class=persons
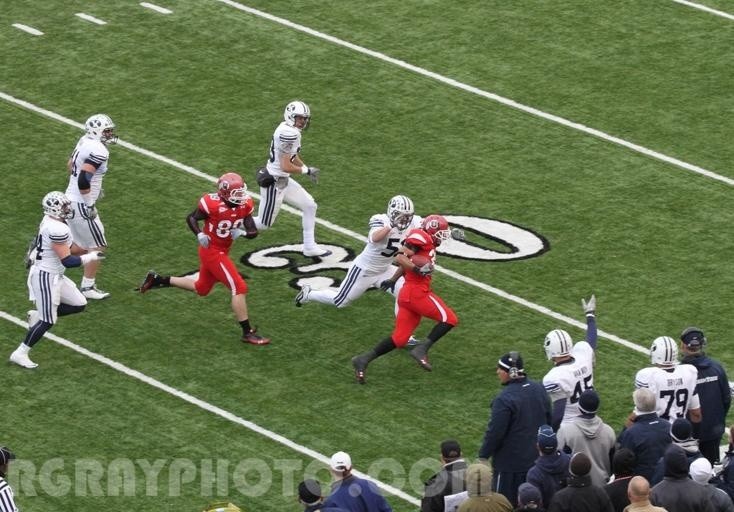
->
[350,214,459,384]
[24,113,118,300]
[237,101,326,257]
[138,173,271,345]
[9,191,106,369]
[294,195,464,346]
[2,291,732,511]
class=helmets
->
[386,195,415,230]
[650,336,679,366]
[544,329,575,361]
[284,100,312,131]
[218,172,248,206]
[85,113,119,146]
[421,214,451,247]
[41,191,75,219]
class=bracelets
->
[389,223,393,229]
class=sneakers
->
[294,283,312,307]
[240,325,271,344]
[10,351,39,369]
[80,282,111,300]
[139,270,158,293]
[351,332,432,385]
[302,246,327,256]
[27,310,39,330]
[23,236,38,269]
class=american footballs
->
[409,256,434,274]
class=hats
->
[330,450,351,472]
[669,418,693,442]
[689,457,712,486]
[680,326,704,348]
[497,354,525,373]
[440,390,599,510]
[298,479,321,503]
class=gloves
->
[84,205,99,220]
[90,250,106,260]
[451,228,466,241]
[197,232,211,248]
[380,279,396,294]
[417,261,434,277]
[388,212,404,228]
[98,188,106,201]
[311,175,319,185]
[229,228,247,240]
[581,294,597,317]
[307,167,320,176]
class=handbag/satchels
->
[255,167,275,187]
